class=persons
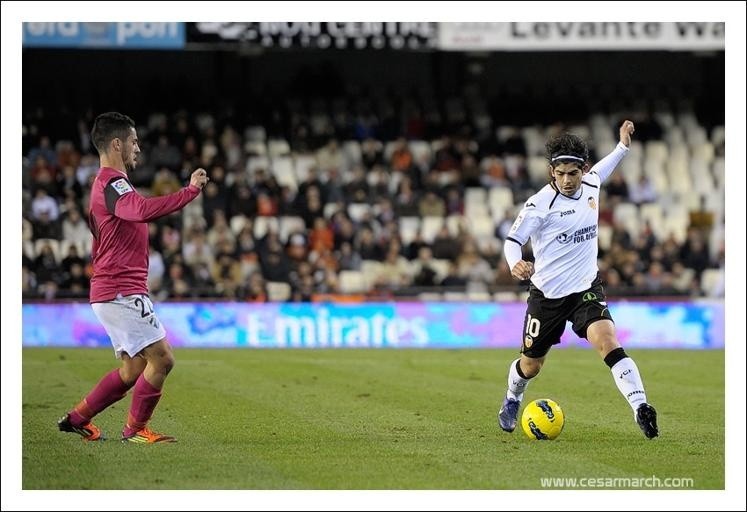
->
[56,112,211,446]
[496,113,661,441]
[22,92,724,304]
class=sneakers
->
[57,413,108,441]
[498,388,520,432]
[636,403,658,439]
[121,427,177,445]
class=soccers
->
[522,398,564,441]
